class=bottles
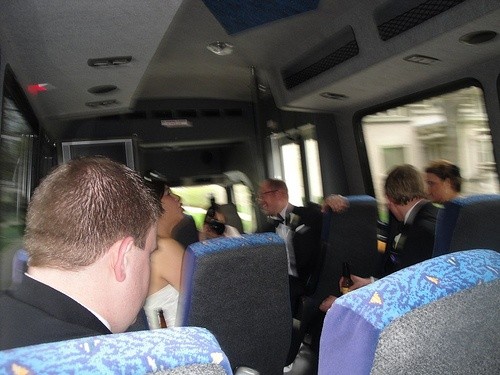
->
[152,307,167,329]
[341,261,353,294]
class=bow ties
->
[272,213,284,228]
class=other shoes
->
[283,343,317,375]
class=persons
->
[1,151,167,351]
[142,161,465,375]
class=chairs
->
[0,326,233,375]
[431,194,500,256]
[174,232,315,375]
[318,247,500,375]
[311,194,377,300]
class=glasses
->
[255,190,278,201]
